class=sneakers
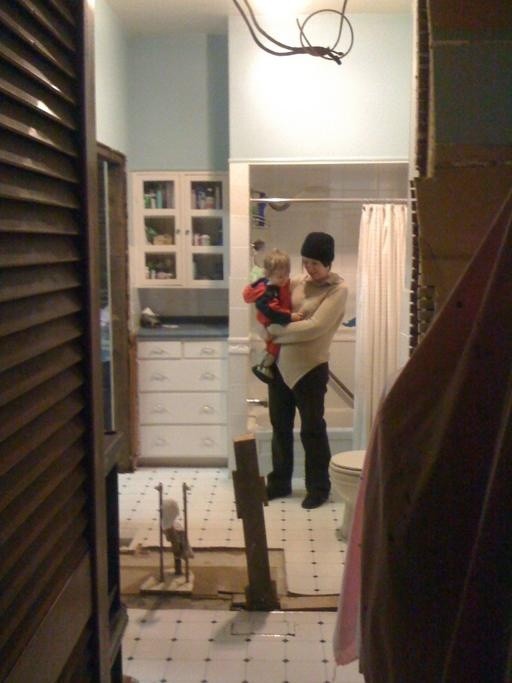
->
[252,365,274,384]
[266,485,328,508]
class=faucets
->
[247,398,269,407]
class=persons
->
[238,247,307,385]
[264,231,352,510]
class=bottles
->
[144,193,156,208]
[206,187,214,209]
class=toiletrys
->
[143,186,215,281]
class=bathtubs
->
[250,406,356,476]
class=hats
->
[301,232,334,268]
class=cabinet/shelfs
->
[134,338,228,468]
[128,169,229,288]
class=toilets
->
[328,450,369,533]
